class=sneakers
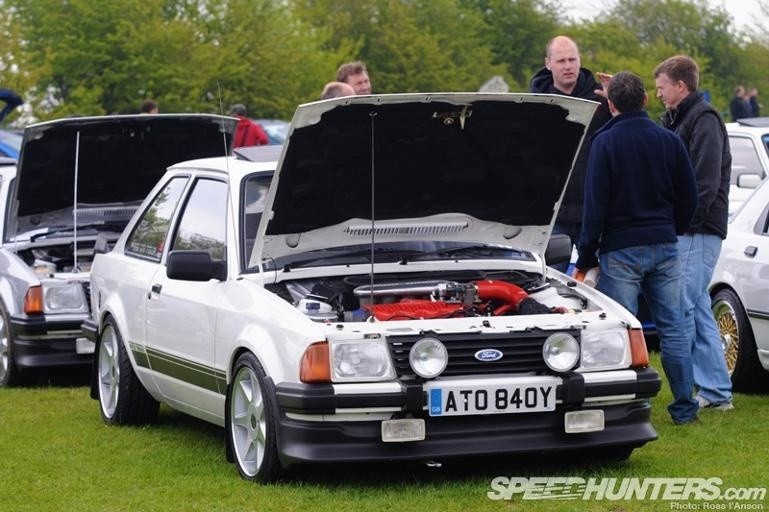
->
[694,393,735,413]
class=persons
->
[140,100,158,114]
[572,71,699,423]
[226,103,269,149]
[321,81,356,100]
[729,85,751,122]
[336,60,371,96]
[529,35,614,278]
[749,88,763,117]
[653,56,735,410]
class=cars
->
[0,87,26,162]
[715,115,769,232]
[80,84,670,471]
[251,118,296,148]
[704,172,768,402]
[0,108,246,392]
